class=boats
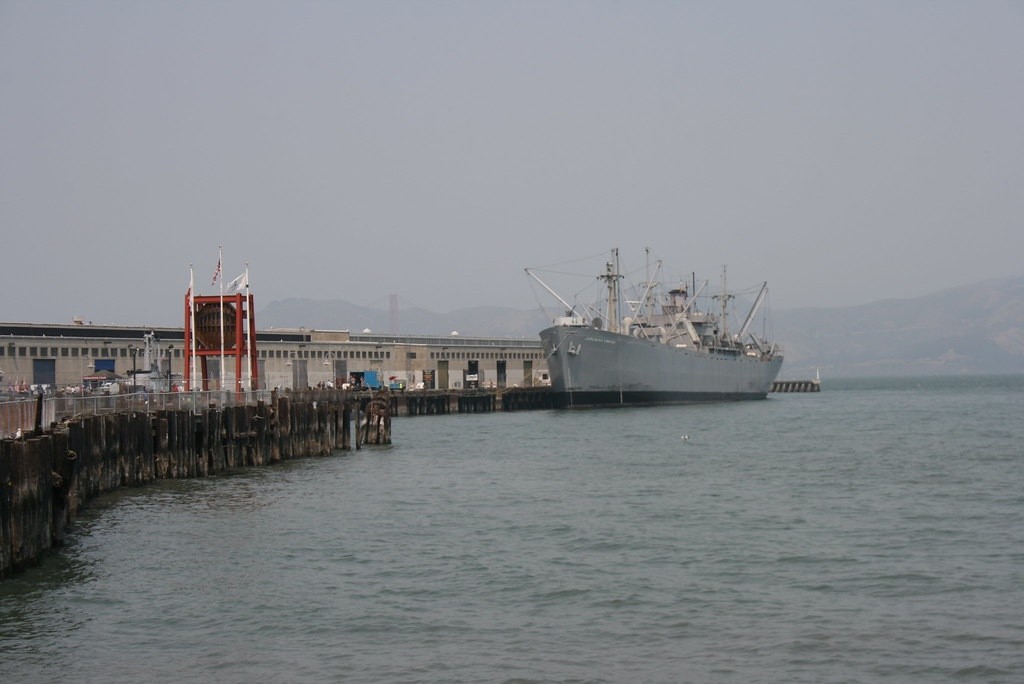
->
[522,248,789,408]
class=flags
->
[185,280,192,312]
[212,258,220,285]
[227,273,247,291]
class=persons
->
[61,385,80,394]
[316,379,334,390]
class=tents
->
[84,369,124,386]
